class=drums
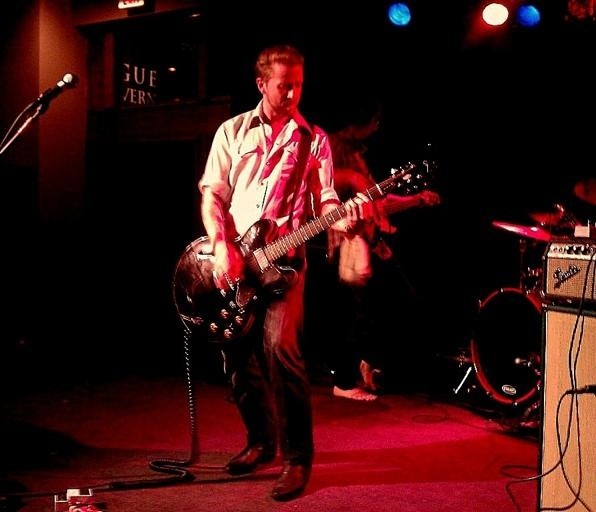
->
[471,287,543,405]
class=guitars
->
[171,160,437,345]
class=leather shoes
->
[271,461,312,500]
[224,436,277,473]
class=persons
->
[321,113,442,406]
[195,42,372,502]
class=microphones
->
[24,73,79,112]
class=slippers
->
[360,362,377,389]
[333,388,378,402]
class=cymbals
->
[489,220,569,245]
[531,212,584,231]
